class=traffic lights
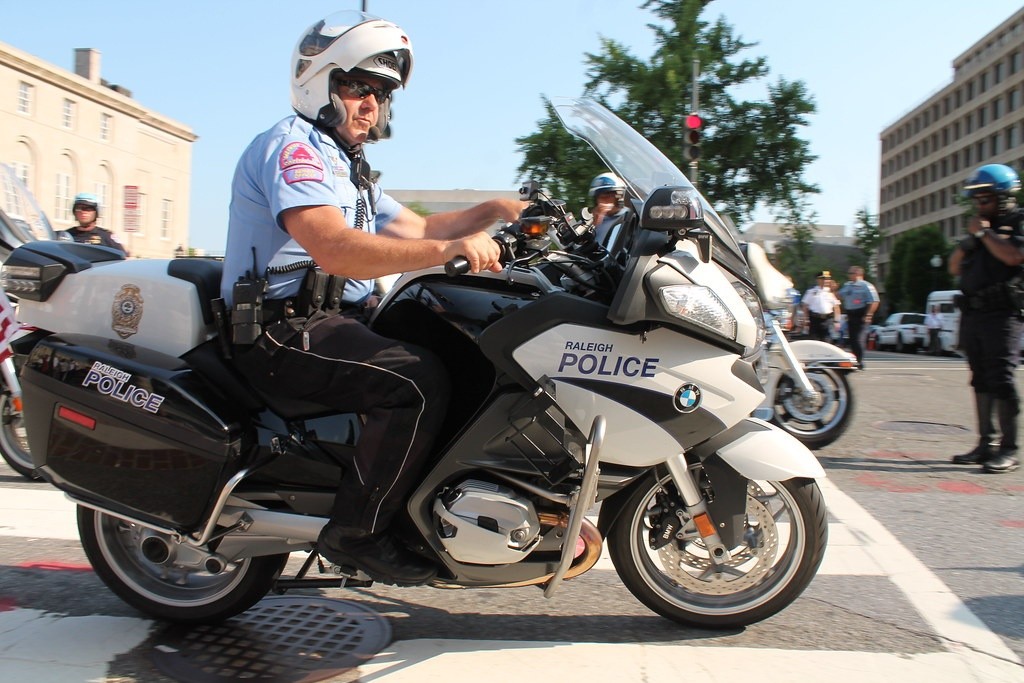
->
[684,111,703,160]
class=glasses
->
[971,196,992,205]
[337,79,387,104]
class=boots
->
[953,393,1002,464]
[984,394,1024,474]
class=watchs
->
[974,227,993,238]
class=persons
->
[782,274,800,341]
[801,270,841,344]
[836,266,881,369]
[586,173,627,230]
[947,163,1024,473]
[221,10,535,588]
[53,193,129,257]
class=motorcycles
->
[1,93,831,638]
[1,163,74,483]
[717,211,860,452]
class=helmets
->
[73,195,102,219]
[589,173,625,208]
[964,164,1022,196]
[291,11,412,140]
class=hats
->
[815,271,832,279]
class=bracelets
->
[832,320,841,323]
[865,313,873,316]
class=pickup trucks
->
[869,311,933,353]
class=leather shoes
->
[319,520,438,588]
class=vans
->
[925,290,964,354]
